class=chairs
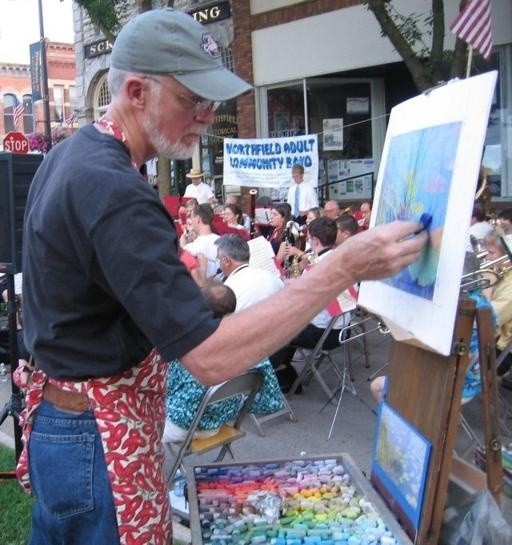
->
[163,370,266,490]
[249,306,512,470]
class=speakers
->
[0,152,43,273]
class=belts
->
[41,384,90,413]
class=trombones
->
[339,250,512,345]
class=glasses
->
[141,75,222,112]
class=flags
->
[449,0,493,63]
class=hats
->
[112,8,253,101]
[186,168,203,178]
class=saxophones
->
[293,248,312,279]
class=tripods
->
[0,273,26,467]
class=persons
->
[11,5,428,545]
